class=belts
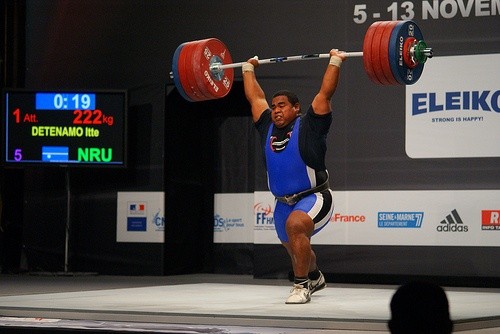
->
[276,179,330,206]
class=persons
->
[242,49,348,305]
[387,279,455,334]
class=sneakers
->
[285,283,311,304]
[309,270,327,295]
